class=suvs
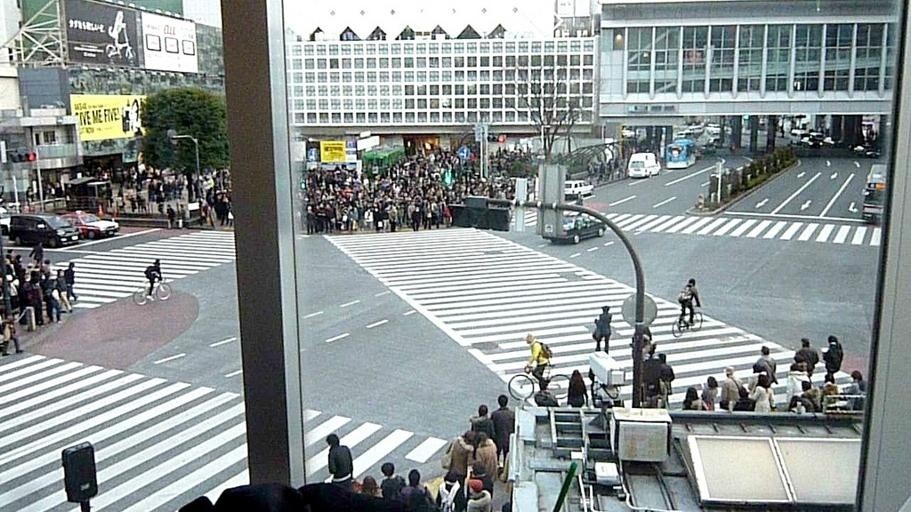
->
[564,180,593,199]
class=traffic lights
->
[10,150,36,162]
[487,134,506,143]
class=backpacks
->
[678,285,694,303]
[535,340,553,359]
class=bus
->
[665,139,695,170]
[863,164,887,225]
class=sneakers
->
[145,293,155,303]
[19,317,62,327]
[3,349,25,357]
[59,295,78,314]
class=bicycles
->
[671,303,703,338]
[132,275,171,306]
[507,364,571,401]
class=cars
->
[0,207,11,236]
[61,210,119,240]
[542,215,606,245]
[791,129,880,159]
[674,123,721,153]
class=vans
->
[627,152,661,179]
[8,214,80,249]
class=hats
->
[723,365,736,376]
[467,477,484,492]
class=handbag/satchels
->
[377,220,383,228]
[440,451,452,471]
[733,399,756,411]
[592,330,603,343]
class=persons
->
[0,241,78,356]
[303,145,531,235]
[682,336,868,415]
[678,278,701,324]
[125,100,142,136]
[638,336,675,409]
[326,433,493,511]
[446,395,515,483]
[144,259,162,301]
[525,334,588,407]
[28,165,234,229]
[595,306,612,353]
